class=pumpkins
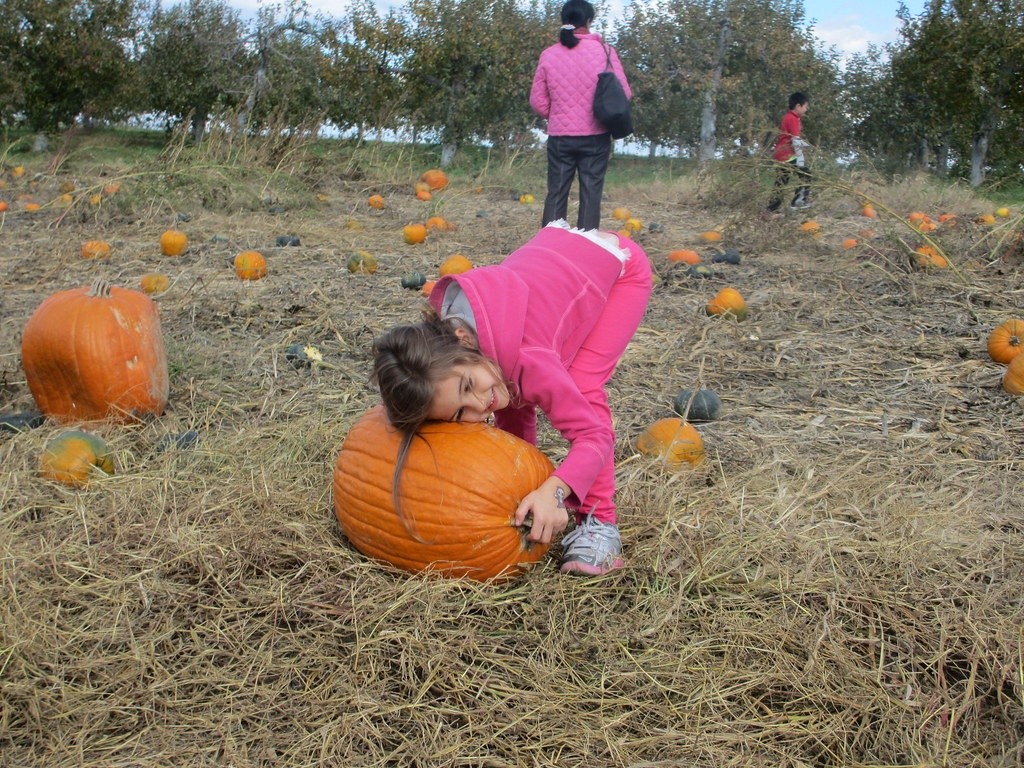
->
[0,168,1024,587]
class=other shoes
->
[769,210,787,220]
[791,201,811,211]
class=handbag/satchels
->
[592,41,635,141]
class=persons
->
[371,219,653,576]
[762,92,810,222]
[530,0,633,232]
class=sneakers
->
[559,500,625,577]
